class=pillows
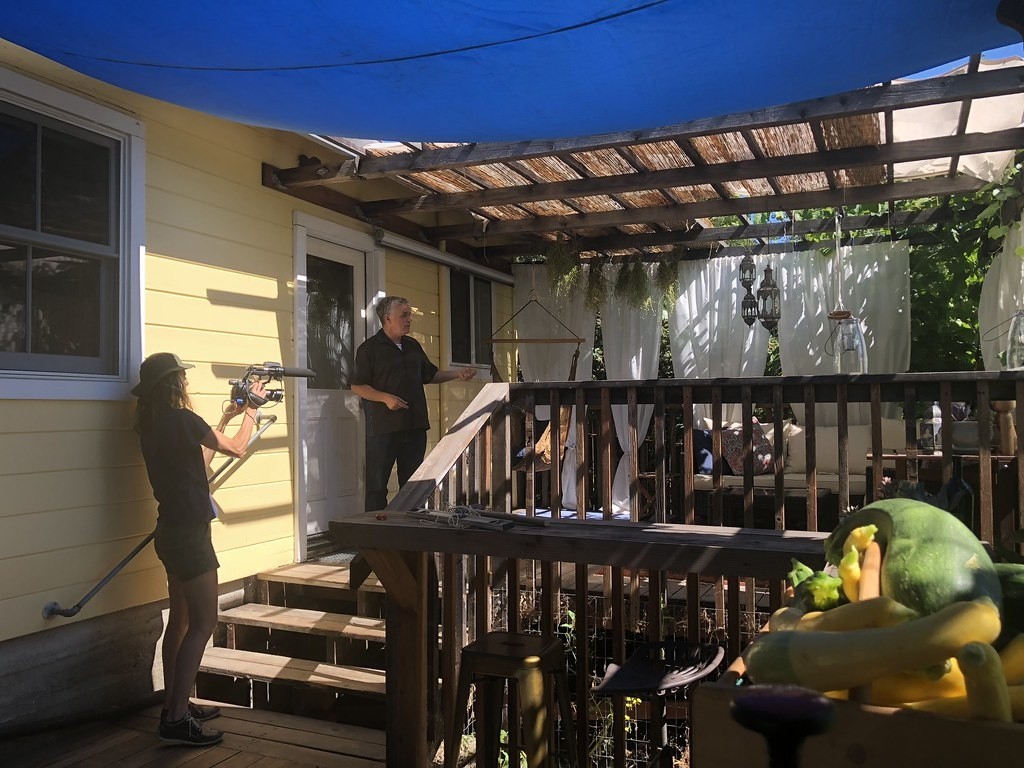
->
[785,424,872,475]
[880,417,920,468]
[691,425,731,475]
[709,415,778,475]
[760,419,793,462]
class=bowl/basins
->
[950,421,992,452]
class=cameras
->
[229,380,284,406]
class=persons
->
[346,296,477,512]
[131,353,269,746]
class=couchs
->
[695,417,932,531]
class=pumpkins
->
[738,498,1024,726]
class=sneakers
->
[160,700,220,720]
[157,711,224,745]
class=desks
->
[637,470,679,522]
[865,451,1019,559]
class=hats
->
[131,353,196,399]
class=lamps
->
[741,287,757,328]
[738,248,760,289]
[758,263,780,332]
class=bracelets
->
[245,411,255,422]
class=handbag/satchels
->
[982,310,1024,369]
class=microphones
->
[281,367,317,377]
